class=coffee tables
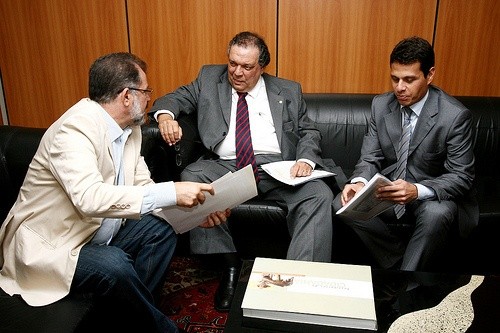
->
[223,259,500,333]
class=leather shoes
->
[214,262,241,312]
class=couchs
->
[0,122,139,333]
[141,91,500,271]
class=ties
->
[235,91,260,186]
[392,105,413,219]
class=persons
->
[332,37,479,272]
[0,52,231,333]
[146,32,347,313]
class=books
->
[335,173,398,226]
[241,258,377,330]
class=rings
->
[304,171,308,172]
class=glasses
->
[117,87,153,97]
